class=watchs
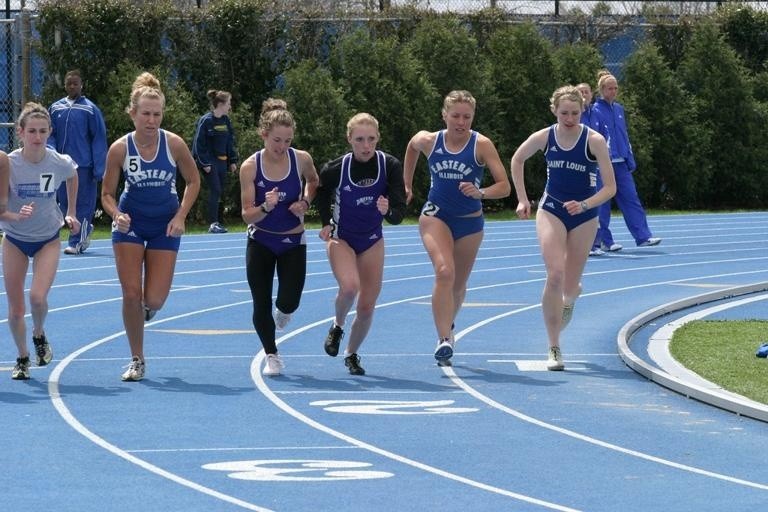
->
[581,200,589,212]
[479,189,486,199]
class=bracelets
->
[259,202,273,215]
[302,199,310,209]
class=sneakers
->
[64,223,94,253]
[557,283,582,332]
[121,360,145,381]
[208,223,227,234]
[263,352,280,376]
[325,322,343,357]
[344,354,365,375]
[32,331,52,366]
[275,309,292,328]
[12,357,30,379]
[548,345,564,371]
[590,237,661,256]
[142,303,157,321]
[435,322,454,366]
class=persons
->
[191,89,238,234]
[0,150,9,215]
[239,97,320,376]
[576,84,622,255]
[589,70,661,251]
[101,71,201,382]
[404,90,511,367]
[510,85,617,371]
[0,101,81,380]
[44,70,107,254]
[315,113,407,375]
